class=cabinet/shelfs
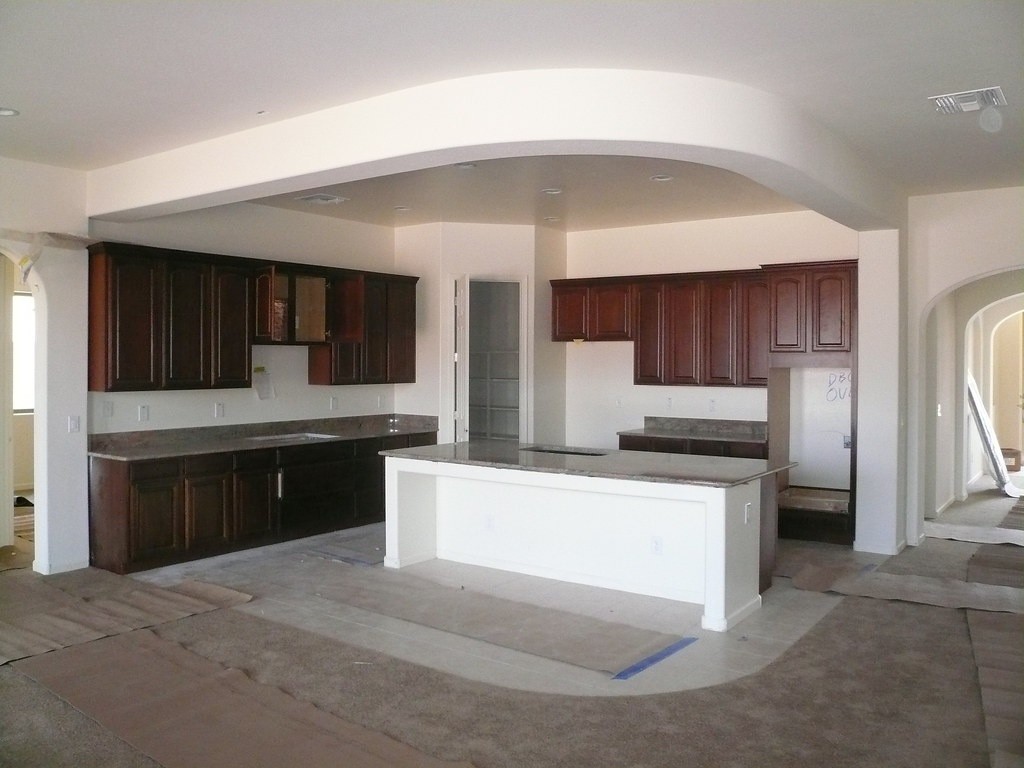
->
[79,239,417,392]
[86,432,437,575]
[618,434,769,461]
[547,257,860,390]
